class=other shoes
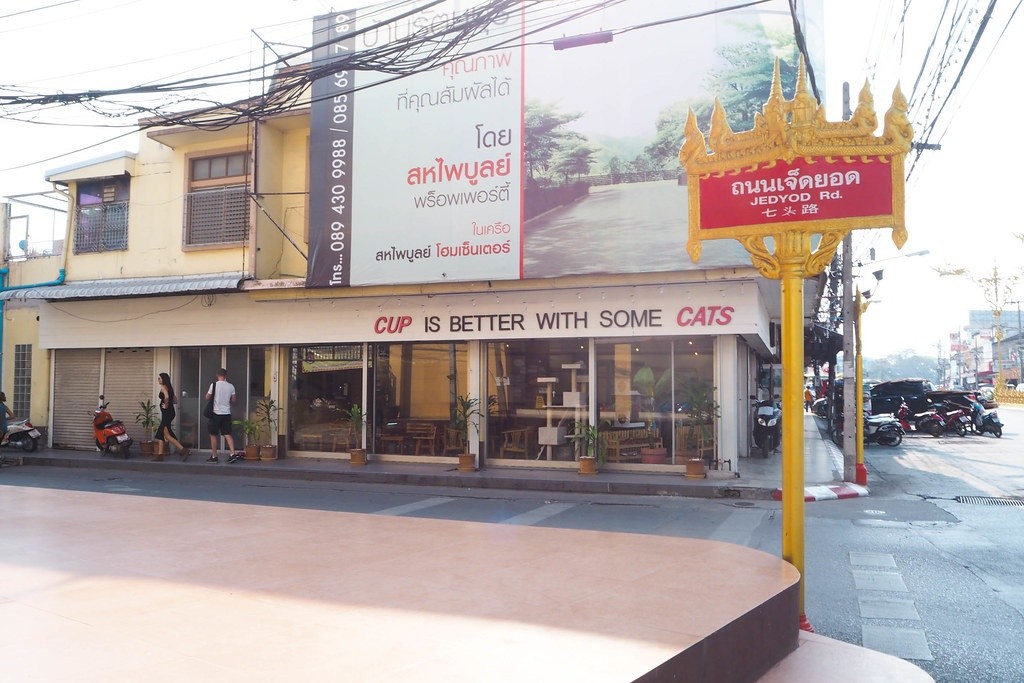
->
[151,453,164,461]
[180,448,191,461]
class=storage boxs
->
[562,391,586,406]
[538,427,567,445]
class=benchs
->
[598,427,663,463]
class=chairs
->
[411,425,438,455]
[301,434,323,451]
[498,425,543,459]
[379,418,405,456]
[329,424,352,453]
[443,425,466,457]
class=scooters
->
[0,417,42,453]
[86,395,133,458]
[750,393,782,458]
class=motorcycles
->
[805,377,1005,447]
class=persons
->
[205,368,239,464]
[0,392,15,443]
[150,373,190,463]
[805,386,814,413]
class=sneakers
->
[225,454,238,463]
[206,456,218,464]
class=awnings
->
[1,272,251,302]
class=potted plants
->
[675,385,723,481]
[568,415,613,475]
[131,398,161,456]
[229,414,265,462]
[253,393,284,462]
[448,391,485,472]
[151,415,184,456]
[626,363,672,464]
[347,403,369,465]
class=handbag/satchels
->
[204,382,216,418]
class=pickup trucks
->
[870,378,975,422]
